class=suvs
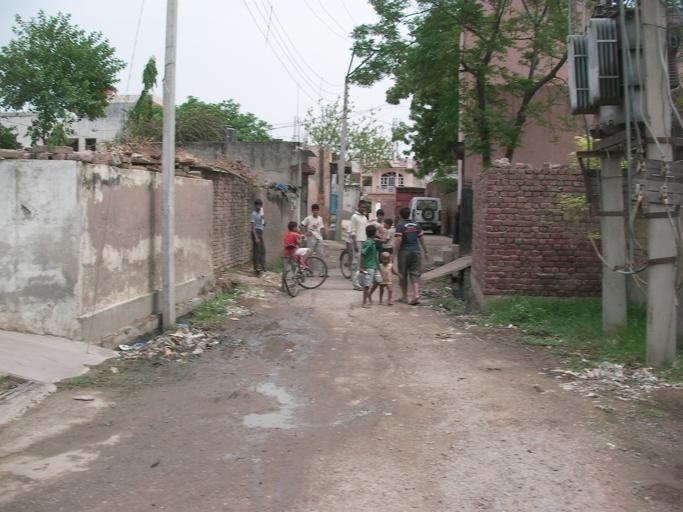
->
[405,197,443,236]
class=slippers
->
[394,298,420,305]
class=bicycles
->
[278,237,394,299]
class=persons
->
[248,198,269,276]
[299,203,330,276]
[277,221,311,293]
[345,199,430,308]
